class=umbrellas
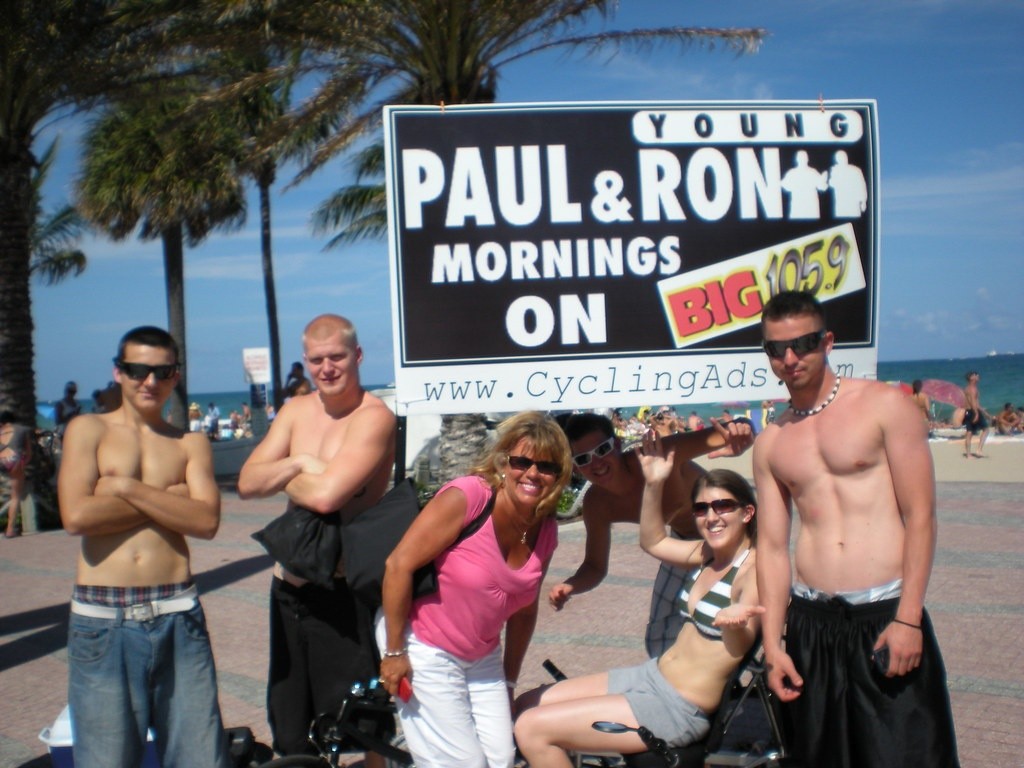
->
[919,379,970,422]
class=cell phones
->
[397,677,413,703]
[874,647,890,673]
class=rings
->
[379,678,385,683]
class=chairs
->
[571,598,789,768]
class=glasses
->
[114,358,179,380]
[505,454,561,474]
[571,437,615,468]
[692,498,747,517]
[761,328,829,359]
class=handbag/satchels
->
[341,476,499,598]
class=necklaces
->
[787,375,842,416]
[500,495,541,544]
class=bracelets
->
[384,647,408,658]
[506,680,517,689]
[893,617,921,630]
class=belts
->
[70,583,197,620]
[271,559,310,586]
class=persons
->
[378,412,573,768]
[752,292,959,768]
[598,377,1024,455]
[0,412,33,539]
[962,370,989,458]
[514,430,767,768]
[58,326,228,768]
[237,315,397,768]
[55,362,316,442]
[548,413,759,666]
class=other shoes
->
[5,530,19,537]
